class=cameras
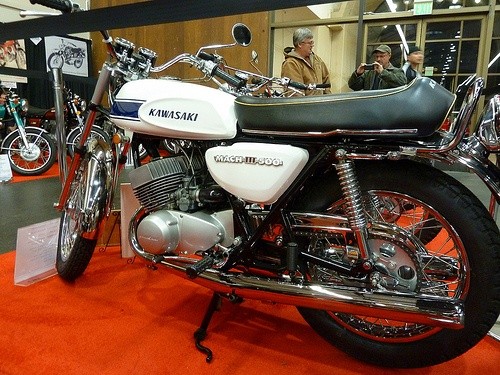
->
[364,64,374,70]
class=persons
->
[402,46,424,85]
[348,45,407,91]
[281,28,331,98]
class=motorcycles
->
[0,83,57,176]
[19,0,500,370]
[18,84,113,164]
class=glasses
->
[301,41,315,45]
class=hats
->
[406,46,421,56]
[372,45,391,53]
